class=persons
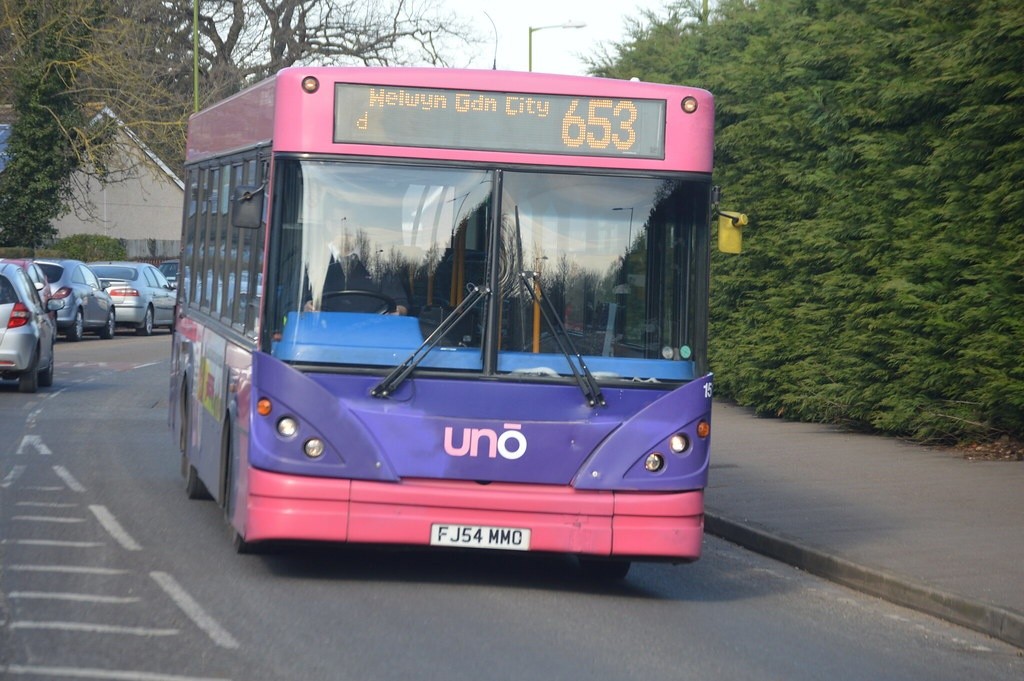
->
[302,205,409,316]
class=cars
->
[162,258,264,326]
[35,258,116,343]
[1,262,66,394]
[85,260,177,337]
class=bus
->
[169,64,750,586]
